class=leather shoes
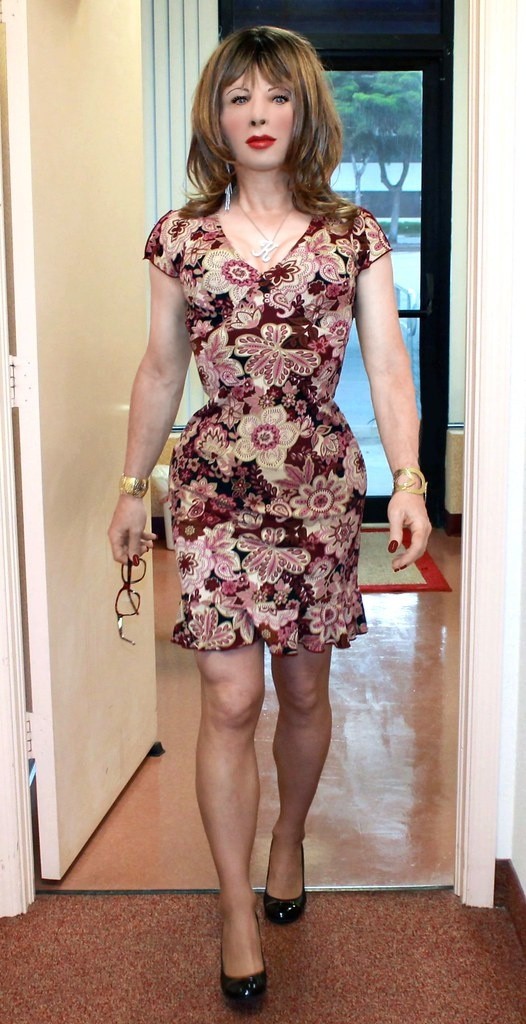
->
[258,833,307,924]
[219,906,267,1011]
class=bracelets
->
[393,468,429,502]
[118,473,150,499]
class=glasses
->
[114,554,147,646]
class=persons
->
[109,25,431,1004]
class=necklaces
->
[237,192,296,263]
